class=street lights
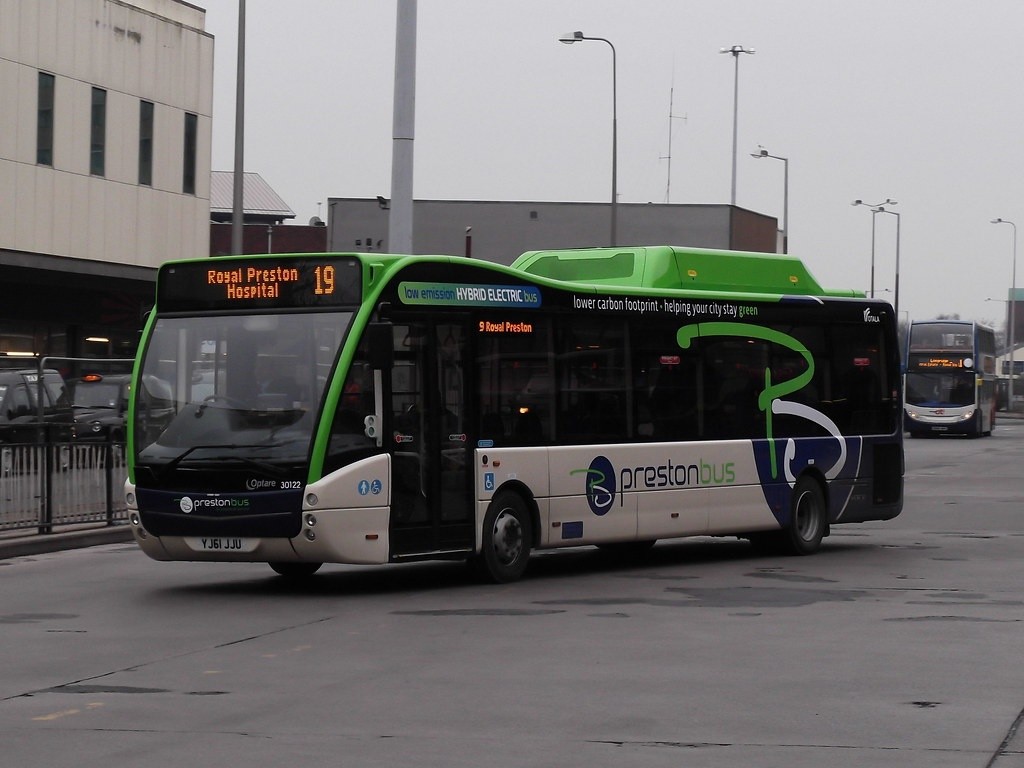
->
[864,289,892,299]
[852,199,900,326]
[869,199,898,300]
[985,298,1009,366]
[560,32,617,247]
[751,150,788,255]
[718,46,756,207]
[990,218,1017,411]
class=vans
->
[0,368,70,476]
[40,373,176,463]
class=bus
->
[901,321,998,438]
[121,245,903,585]
